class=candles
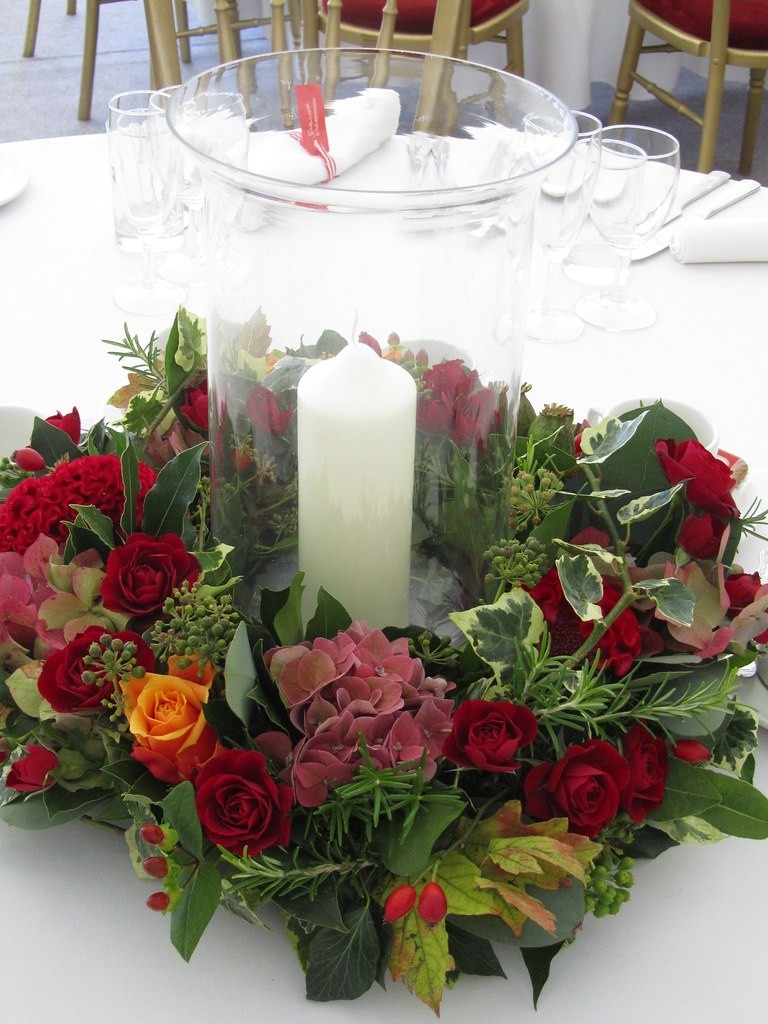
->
[292,341,421,631]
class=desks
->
[1,133,768,1020]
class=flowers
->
[0,325,768,995]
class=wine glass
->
[576,121,691,333]
[104,86,274,326]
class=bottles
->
[513,109,606,343]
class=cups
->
[158,44,582,658]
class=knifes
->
[629,168,763,263]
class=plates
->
[0,145,31,209]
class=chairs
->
[22,3,306,143]
[610,0,767,182]
[305,0,533,146]
